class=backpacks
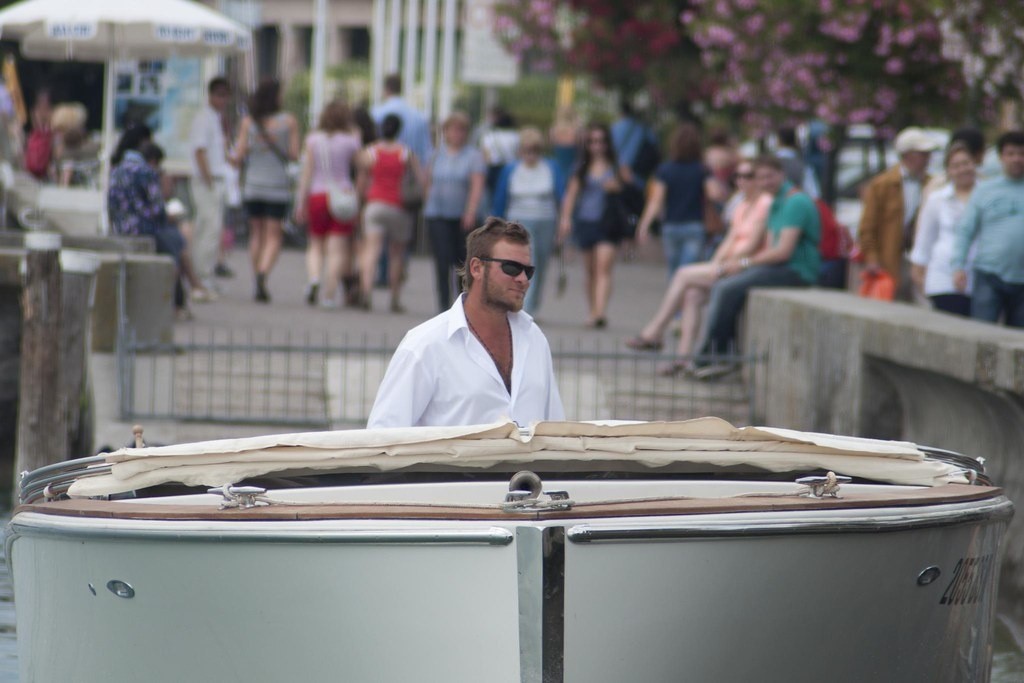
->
[785,188,849,291]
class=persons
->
[0,68,1024,380]
[363,215,566,430]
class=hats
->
[895,125,941,152]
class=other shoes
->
[388,301,405,311]
[694,361,733,381]
[661,361,687,375]
[308,283,322,304]
[590,315,607,328]
[626,333,664,350]
[256,275,270,304]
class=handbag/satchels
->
[325,183,359,221]
[284,154,303,180]
[859,268,899,301]
[609,191,661,238]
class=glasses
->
[733,171,754,180]
[478,256,535,280]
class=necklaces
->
[461,313,514,397]
